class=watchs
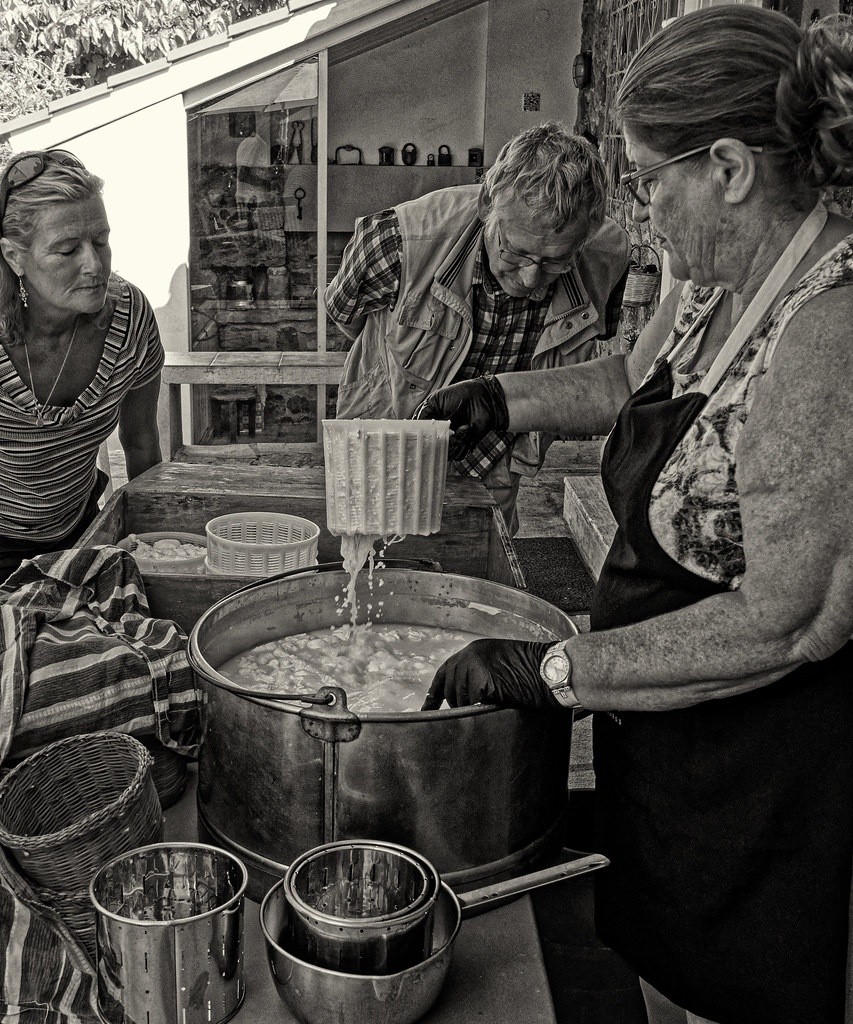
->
[541,640,585,711]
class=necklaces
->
[24,319,78,427]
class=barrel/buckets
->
[184,557,582,885]
[89,840,248,1024]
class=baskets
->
[115,531,208,571]
[0,730,166,973]
[321,416,450,536]
[621,244,662,308]
[205,511,320,578]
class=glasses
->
[0,148,86,224]
[489,195,587,274]
[619,142,764,208]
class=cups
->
[378,145,395,166]
[467,147,482,167]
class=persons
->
[323,121,632,538]
[417,6,853,1024]
[0,151,165,581]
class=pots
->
[258,853,612,1024]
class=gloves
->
[411,373,510,462]
[421,638,562,713]
